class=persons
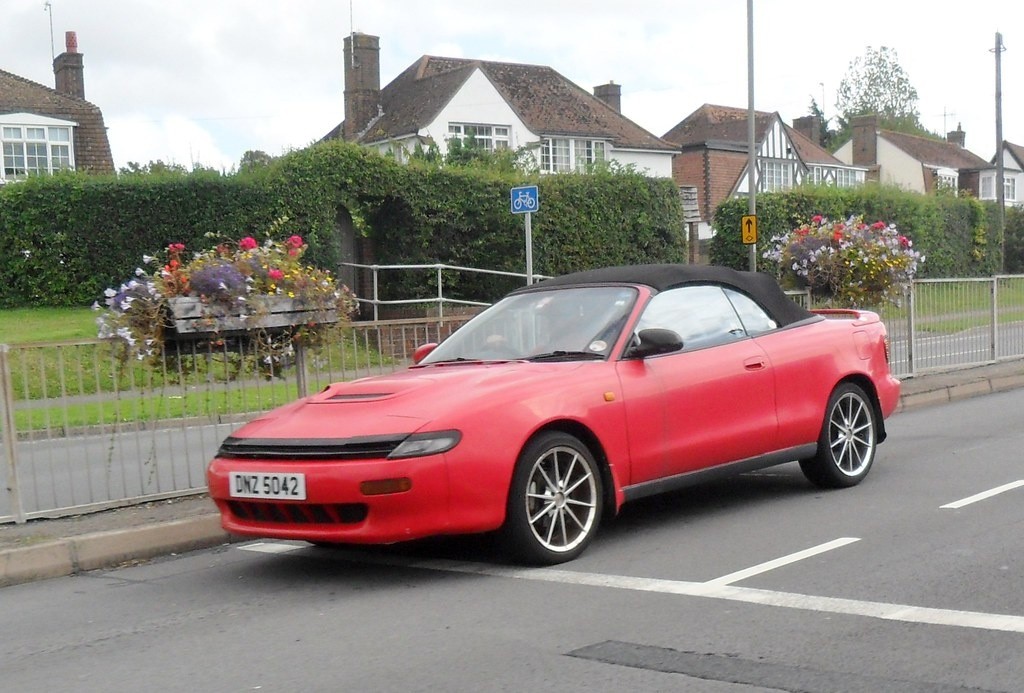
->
[485,297,582,360]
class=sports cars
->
[205,263,904,568]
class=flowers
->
[92,231,360,381]
[756,211,926,312]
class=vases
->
[810,276,883,297]
[166,290,338,334]
